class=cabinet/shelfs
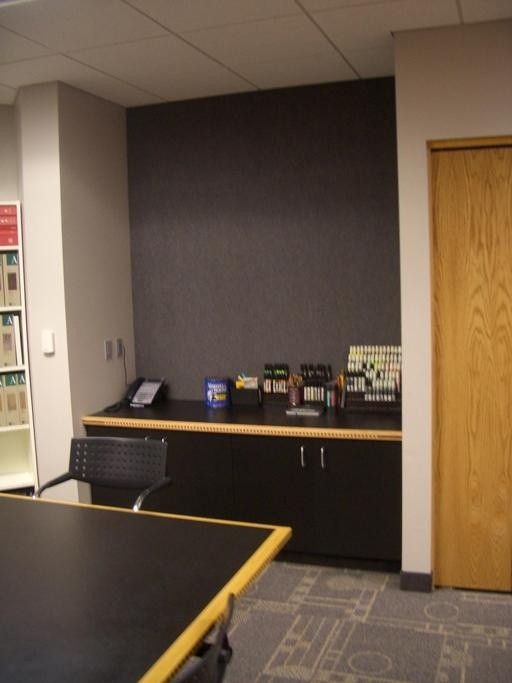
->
[0,200,38,493]
[235,424,401,571]
[83,416,233,520]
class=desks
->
[0,486,294,682]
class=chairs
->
[35,436,173,514]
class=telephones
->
[123,377,165,411]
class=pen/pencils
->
[266,345,401,407]
